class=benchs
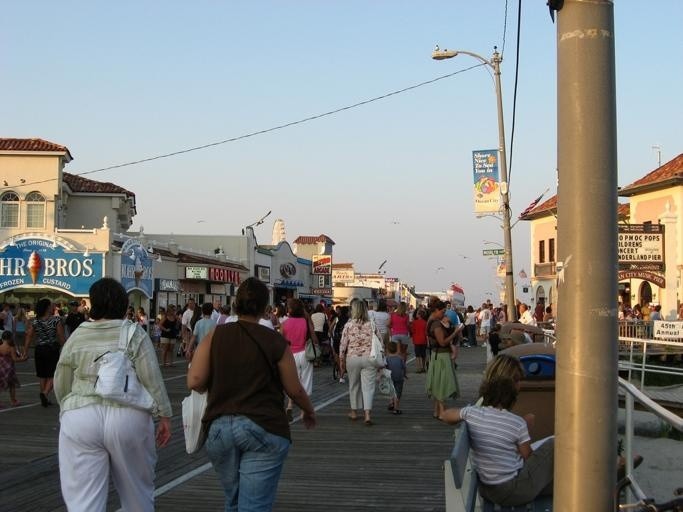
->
[442,413,535,511]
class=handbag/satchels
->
[94,351,157,412]
[323,322,329,335]
[376,369,397,400]
[304,339,321,362]
[370,333,387,369]
[465,318,472,326]
[181,390,206,454]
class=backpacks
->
[484,311,490,320]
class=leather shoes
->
[348,413,358,420]
[365,418,371,425]
[616,453,643,480]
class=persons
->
[261,295,467,426]
[439,375,559,509]
[473,352,535,431]
[127,297,238,368]
[615,454,644,484]
[618,300,664,341]
[186,277,319,512]
[2,298,91,407]
[53,277,173,510]
[464,298,556,353]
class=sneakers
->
[40,391,49,407]
[9,400,22,407]
[481,343,486,348]
[339,377,346,383]
[300,414,304,422]
[286,406,294,422]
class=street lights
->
[430,45,520,331]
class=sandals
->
[391,409,403,414]
[387,404,394,411]
